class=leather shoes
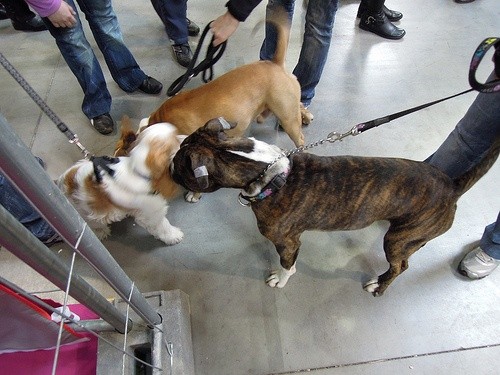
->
[90,112,113,135]
[358,7,406,40]
[357,5,404,22]
[137,75,162,94]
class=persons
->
[421,69,500,281]
[357,0,406,40]
[0,0,164,135]
[151,0,200,67]
[209,0,338,127]
[0,156,64,248]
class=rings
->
[60,23,65,26]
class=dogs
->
[54,7,314,245]
[169,118,500,296]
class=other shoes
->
[11,15,49,32]
[0,12,9,20]
[43,233,63,248]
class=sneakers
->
[458,247,500,280]
[170,38,194,67]
[186,17,200,36]
